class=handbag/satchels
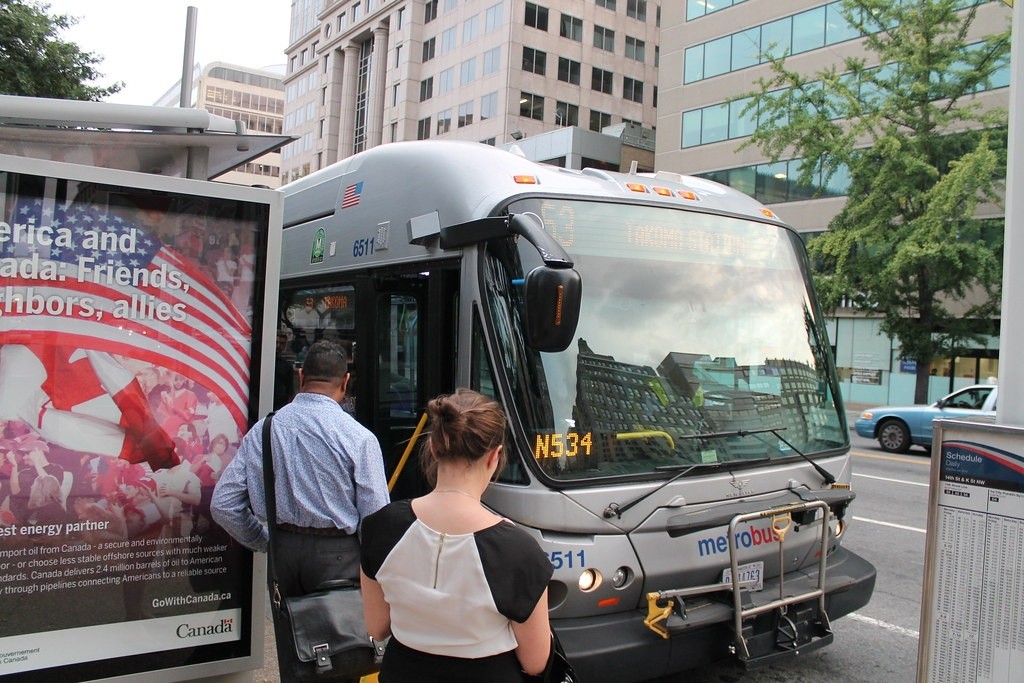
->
[280,579,392,683]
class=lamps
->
[510,130,523,140]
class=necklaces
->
[433,489,480,503]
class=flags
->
[0,174,252,472]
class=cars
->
[853,376,1001,454]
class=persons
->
[211,339,391,683]
[0,217,256,639]
[360,387,579,683]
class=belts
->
[281,523,347,537]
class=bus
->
[266,137,877,683]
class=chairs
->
[975,394,988,409]
